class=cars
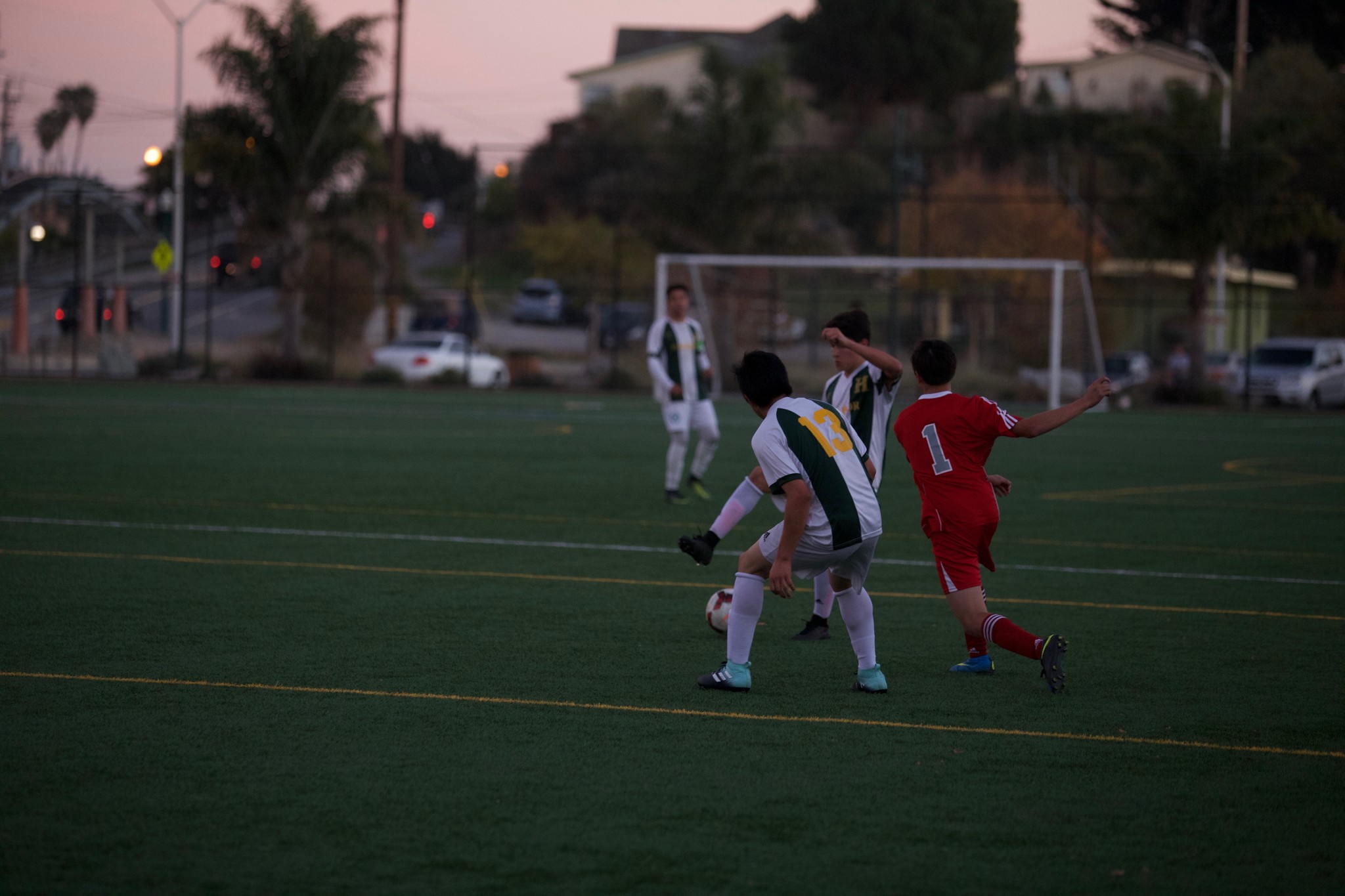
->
[510,278,565,321]
[1197,352,1246,390]
[408,294,478,343]
[598,300,653,349]
[369,334,509,389]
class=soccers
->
[705,588,734,635]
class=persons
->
[1167,344,1191,378]
[893,337,1113,692]
[696,348,888,695]
[645,284,722,504]
[678,310,904,640]
[1101,340,1131,380]
[410,289,482,343]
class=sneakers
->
[947,654,995,676]
[677,533,714,567]
[1039,634,1070,695]
[790,621,830,642]
[848,664,888,695]
[696,658,752,694]
[663,488,690,506]
[686,473,711,501]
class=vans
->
[210,239,285,284]
[1086,351,1150,394]
[1238,336,1345,407]
[55,284,137,332]
